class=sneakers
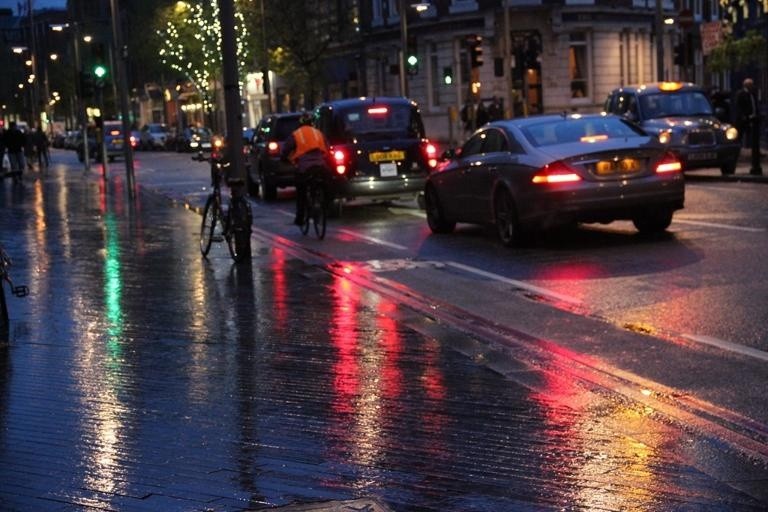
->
[294,216,305,225]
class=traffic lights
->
[467,36,484,70]
[94,65,107,81]
[442,67,453,87]
[405,39,418,78]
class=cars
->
[425,112,683,251]
[50,123,217,162]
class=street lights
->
[12,21,90,162]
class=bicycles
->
[292,167,328,239]
[189,154,249,264]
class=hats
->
[300,113,316,124]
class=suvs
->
[242,94,432,203]
[605,81,740,176]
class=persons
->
[459,88,526,140]
[0,122,50,181]
[283,123,329,223]
